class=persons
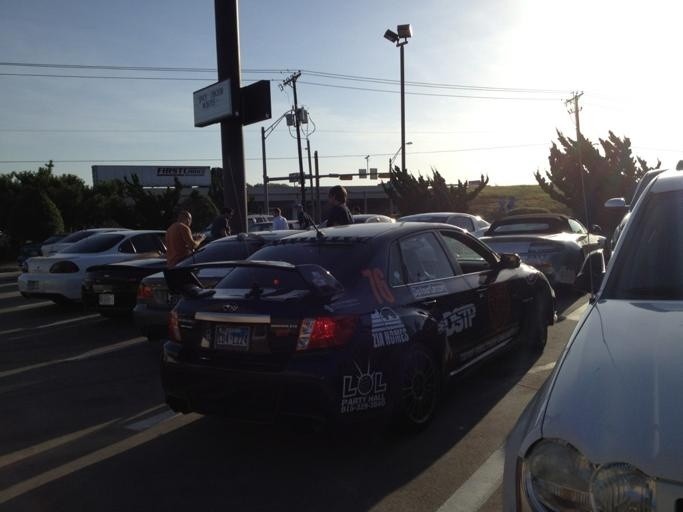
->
[164,210,208,267]
[508,195,517,209]
[271,208,290,232]
[326,185,361,227]
[212,207,237,238]
[499,194,506,214]
[295,203,312,231]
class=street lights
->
[383,23,413,175]
[389,141,413,216]
